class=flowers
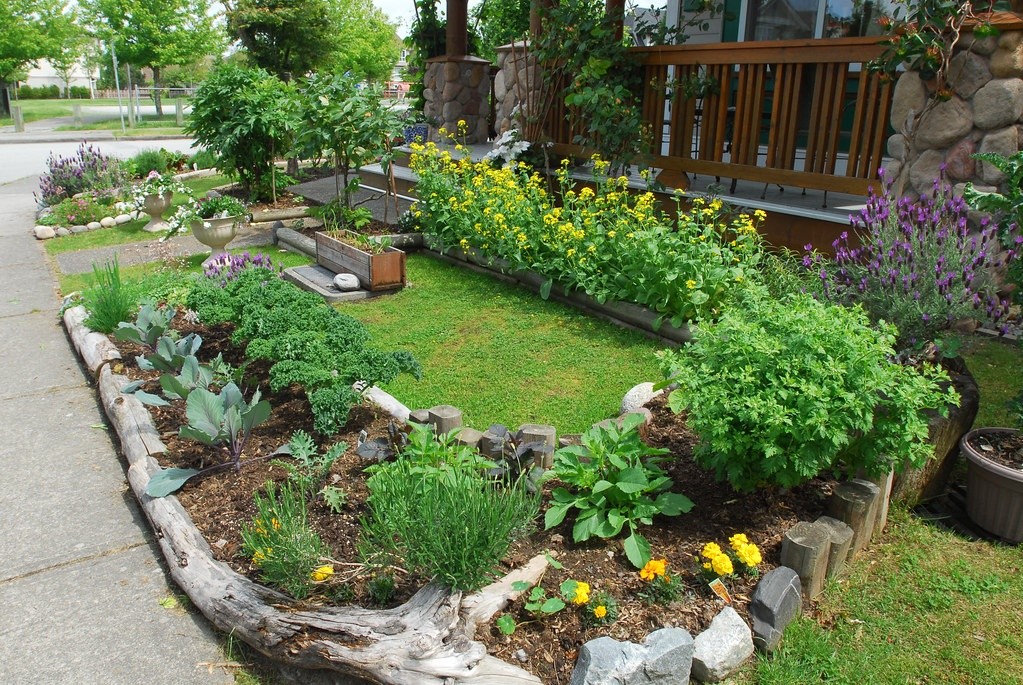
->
[157,186,255,244]
[800,161,1023,362]
[127,169,193,220]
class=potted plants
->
[401,110,441,148]
[960,151,1023,544]
[315,177,406,291]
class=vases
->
[914,349,979,504]
[190,214,240,267]
[141,191,173,232]
